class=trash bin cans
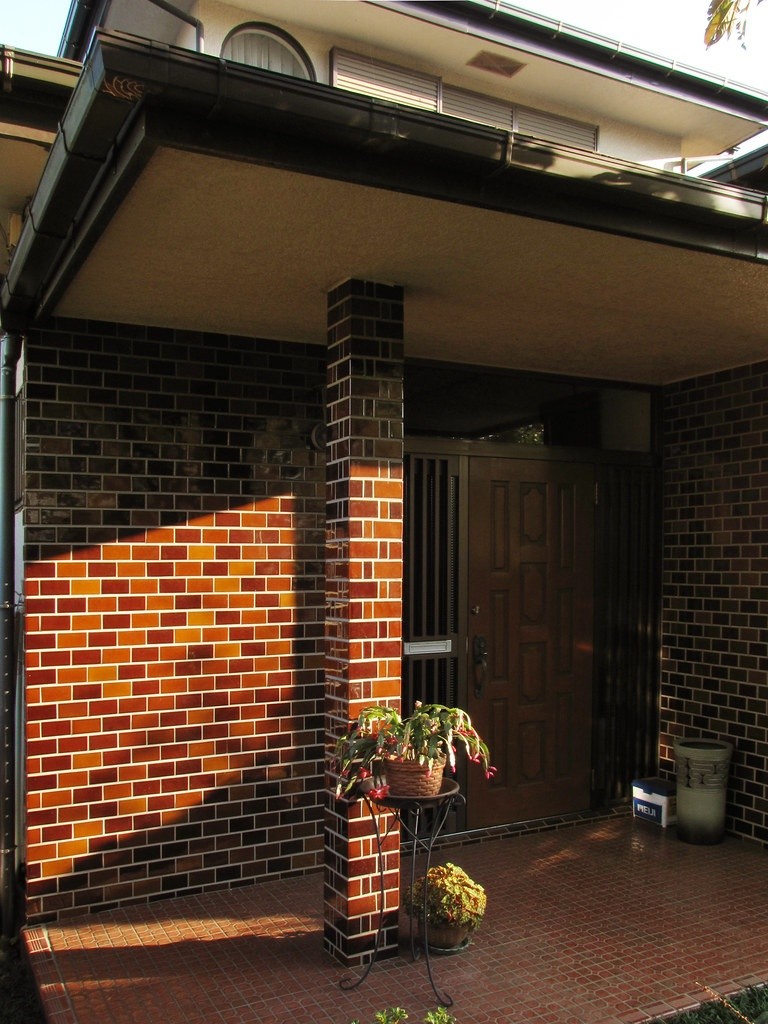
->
[674,738,732,845]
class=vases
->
[415,916,470,948]
[380,753,448,797]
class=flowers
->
[398,861,489,934]
[323,697,498,801]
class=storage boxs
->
[632,777,678,828]
